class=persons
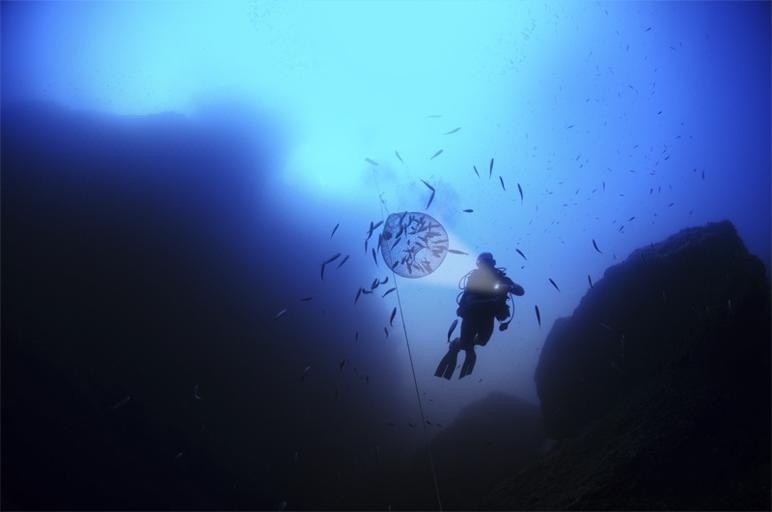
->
[433,253,524,380]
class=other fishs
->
[235,125,542,432]
[544,7,768,334]
